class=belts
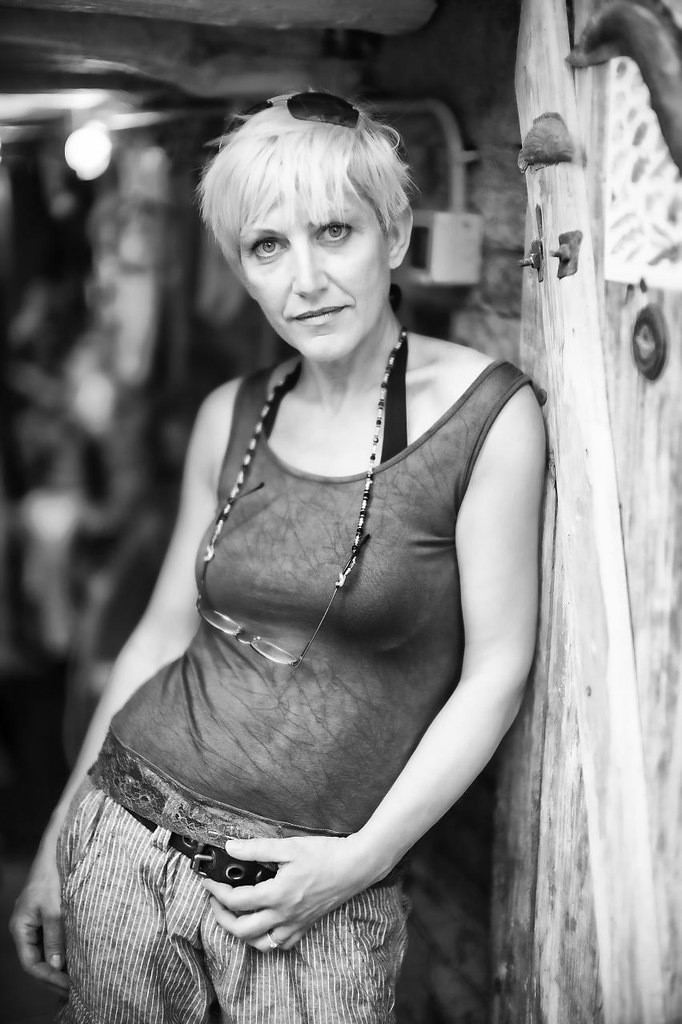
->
[120,801,279,887]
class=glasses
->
[195,482,373,668]
[219,91,372,153]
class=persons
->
[7,91,544,1024]
